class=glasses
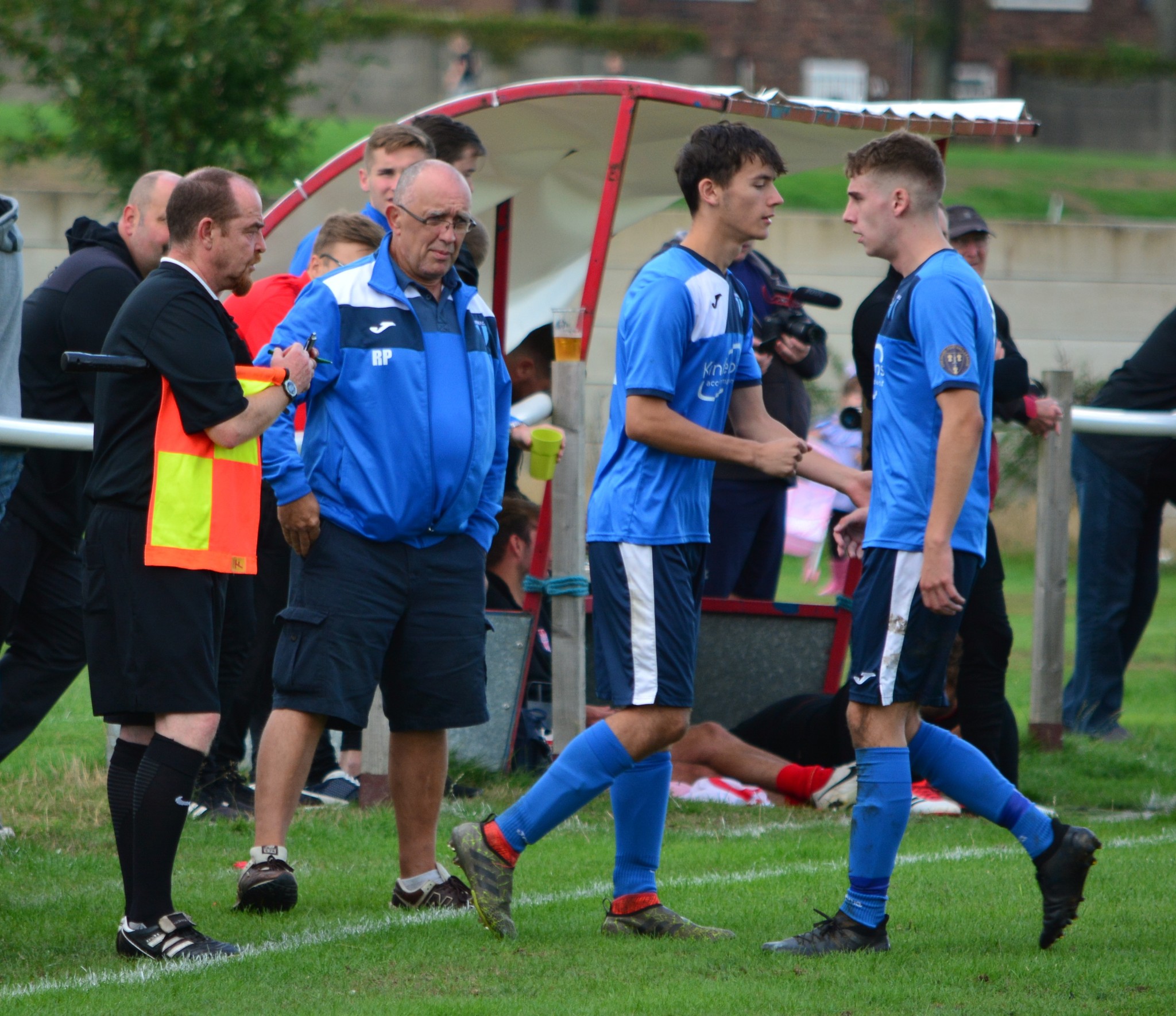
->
[398,207,476,234]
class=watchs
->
[282,380,297,405]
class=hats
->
[947,206,996,239]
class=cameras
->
[755,294,828,354]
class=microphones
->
[772,284,841,307]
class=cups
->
[552,307,586,361]
[529,428,563,482]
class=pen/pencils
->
[267,349,332,363]
[306,332,317,351]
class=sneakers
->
[910,780,963,815]
[114,911,242,959]
[190,778,255,822]
[1035,817,1101,950]
[389,864,474,911]
[602,897,734,942]
[230,845,299,913]
[299,767,359,805]
[762,907,890,954]
[445,811,517,940]
[814,760,857,812]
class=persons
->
[449,121,871,941]
[1063,307,1175,742]
[764,127,1103,955]
[0,114,1059,964]
